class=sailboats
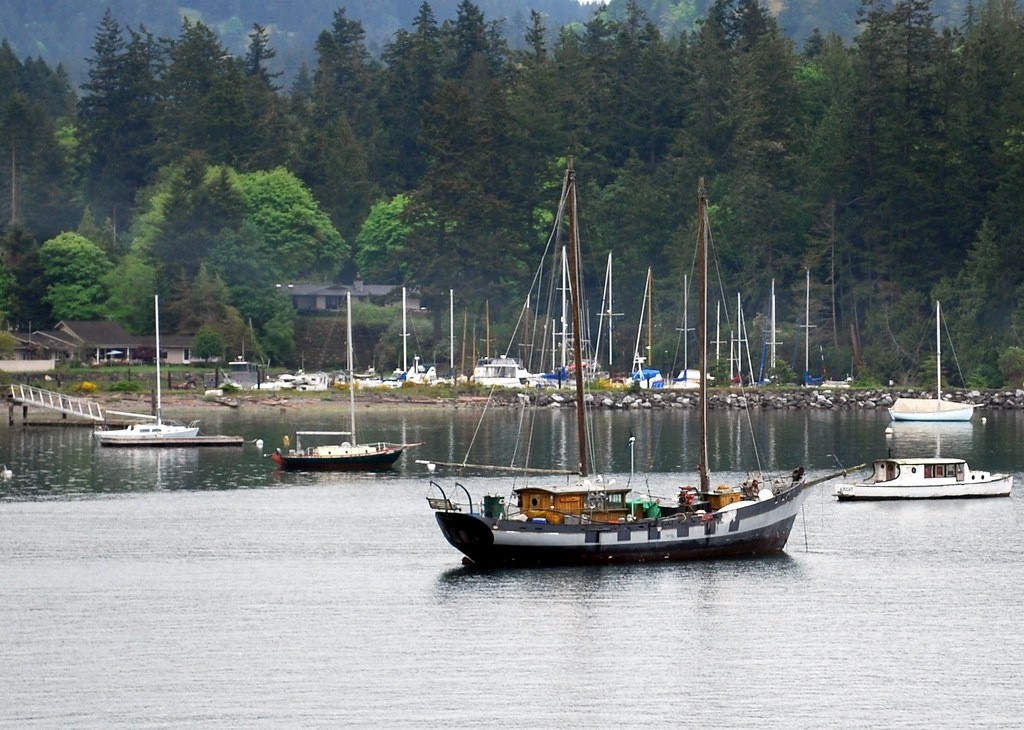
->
[886,298,984,423]
[252,245,853,389]
[261,289,428,472]
[426,151,870,566]
[93,293,245,448]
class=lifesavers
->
[283,434,290,448]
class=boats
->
[832,456,1014,499]
